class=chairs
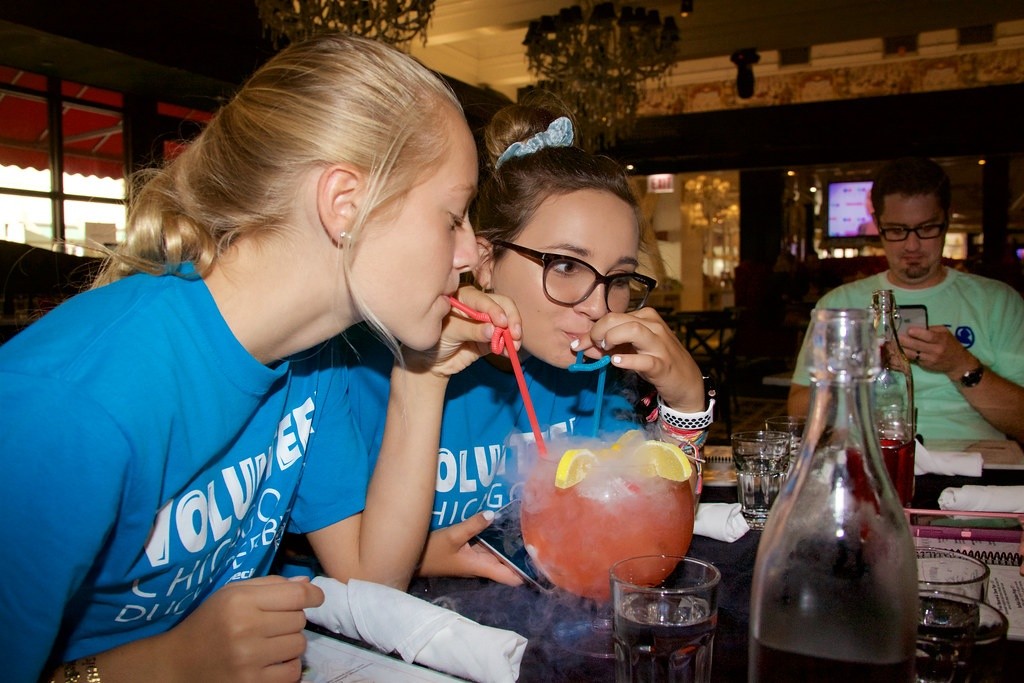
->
[655,307,739,444]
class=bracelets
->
[644,406,711,495]
[47,658,101,683]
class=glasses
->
[875,215,949,242]
[488,236,659,314]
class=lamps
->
[730,49,760,100]
[254,0,436,55]
[522,0,681,153]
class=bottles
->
[868,290,915,509]
[750,307,917,683]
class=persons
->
[0,33,522,683]
[787,155,1024,446]
[344,91,720,589]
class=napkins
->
[938,485,1024,521]
[302,576,528,683]
[692,503,750,544]
[915,438,985,478]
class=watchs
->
[951,362,985,388]
[656,376,718,431]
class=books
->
[912,532,1024,640]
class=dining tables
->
[298,435,1024,683]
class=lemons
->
[612,429,645,455]
[554,449,598,490]
[632,440,692,482]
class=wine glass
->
[521,431,693,658]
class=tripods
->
[821,177,883,243]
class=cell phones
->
[473,500,562,595]
[894,305,929,339]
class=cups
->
[731,431,792,530]
[765,416,808,479]
[916,545,1009,683]
[610,553,721,683]
